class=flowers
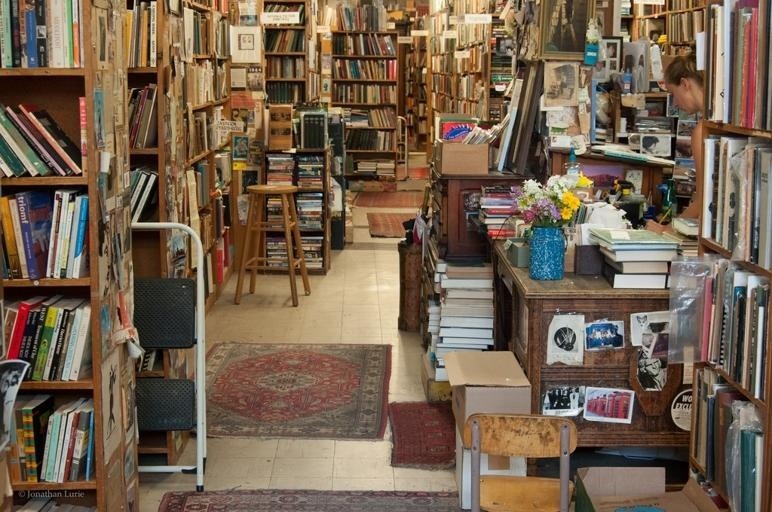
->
[512,175,580,228]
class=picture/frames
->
[504,61,544,176]
[538,0,596,61]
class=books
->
[325,1,395,30]
[0,94,88,183]
[294,234,323,269]
[489,88,506,122]
[432,74,452,95]
[490,53,512,86]
[345,129,394,151]
[5,393,96,485]
[699,251,769,404]
[215,150,232,190]
[441,0,489,16]
[215,18,228,59]
[429,35,455,55]
[368,107,395,127]
[212,107,225,148]
[664,230,699,258]
[431,55,452,75]
[671,215,700,234]
[480,185,529,241]
[639,0,665,17]
[687,367,764,512]
[456,48,484,74]
[266,198,292,228]
[266,29,305,52]
[704,0,772,134]
[345,153,354,174]
[425,260,496,385]
[428,12,448,36]
[457,74,484,100]
[296,155,323,187]
[491,17,510,50]
[135,346,157,374]
[0,186,89,280]
[124,1,160,71]
[0,292,91,382]
[185,111,209,159]
[184,159,210,213]
[456,23,488,48]
[266,3,305,26]
[330,33,396,56]
[197,207,224,253]
[669,0,700,11]
[126,81,158,150]
[294,192,323,231]
[638,18,667,46]
[193,9,210,57]
[699,131,772,272]
[266,232,289,268]
[456,100,483,117]
[264,152,295,187]
[217,62,228,100]
[195,0,220,12]
[266,81,303,104]
[202,238,232,300]
[265,56,305,79]
[130,165,160,226]
[332,82,396,105]
[431,93,451,112]
[588,227,677,290]
[668,11,703,43]
[332,58,397,81]
[376,162,395,176]
[303,114,325,150]
[0,0,84,72]
[401,22,429,136]
[357,161,377,173]
[188,58,215,106]
[342,107,369,127]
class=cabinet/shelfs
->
[125,0,237,475]
[595,0,723,158]
[318,0,400,193]
[686,0,772,512]
[386,1,430,152]
[261,149,331,275]
[426,0,514,164]
[264,0,320,107]
[1,0,141,511]
[296,111,345,251]
[227,0,267,270]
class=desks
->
[432,160,537,262]
[465,212,694,478]
[542,145,675,223]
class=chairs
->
[463,414,577,511]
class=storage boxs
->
[458,424,526,511]
[433,138,488,175]
[574,467,721,512]
[442,351,531,450]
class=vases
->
[528,227,565,280]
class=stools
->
[234,185,311,307]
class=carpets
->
[188,342,391,441]
[352,191,425,208]
[387,401,456,471]
[156,487,460,512]
[367,212,416,237]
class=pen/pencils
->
[659,208,673,226]
